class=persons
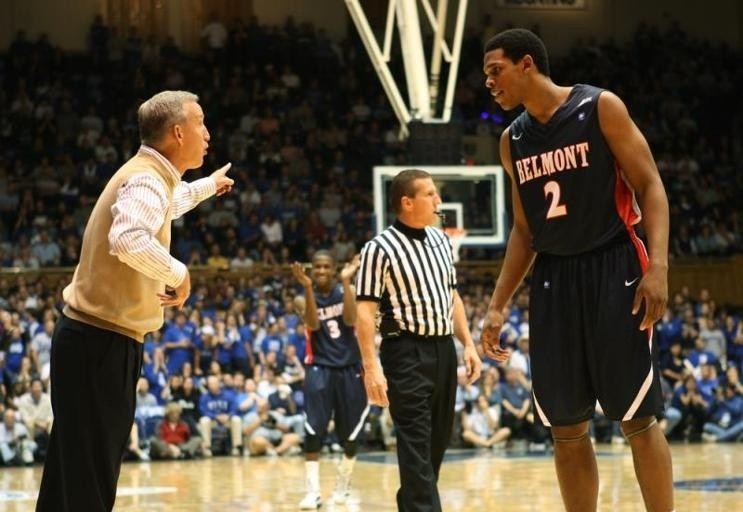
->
[291,247,370,512]
[36,87,237,512]
[1,13,743,462]
[477,24,676,512]
[352,167,483,511]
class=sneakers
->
[300,492,321,510]
[334,466,353,503]
